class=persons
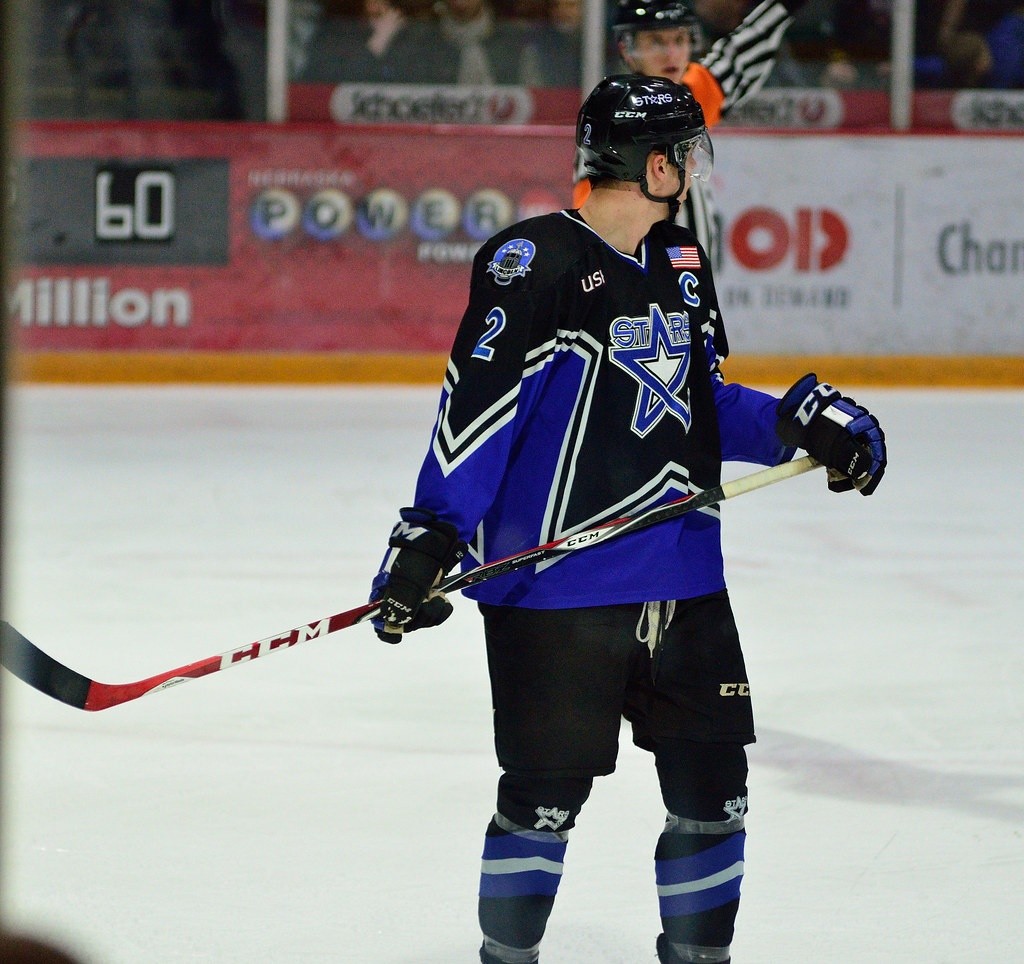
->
[610,0,800,257]
[300,0,997,93]
[369,72,886,964]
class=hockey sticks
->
[0,451,825,716]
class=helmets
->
[611,0,694,41]
[574,74,706,183]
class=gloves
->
[369,506,466,644]
[775,372,887,495]
